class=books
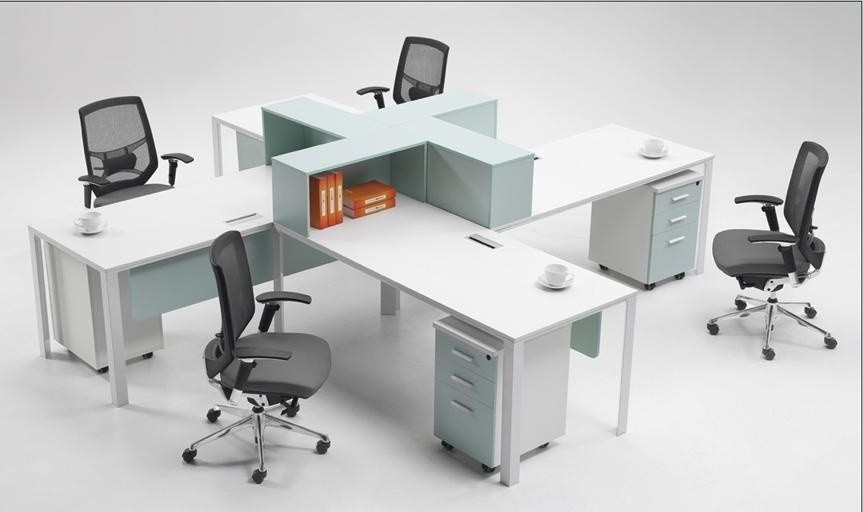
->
[328,170,343,224]
[343,197,396,218]
[343,180,395,209]
[310,174,328,229]
[321,172,336,227]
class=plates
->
[71,219,107,234]
[537,272,576,290]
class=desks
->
[211,85,498,176]
[426,122,715,293]
[25,162,401,408]
[272,186,637,486]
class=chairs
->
[356,36,451,112]
[182,231,331,483]
[78,94,193,209]
[708,142,839,362]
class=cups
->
[74,211,102,231]
[545,263,574,285]
